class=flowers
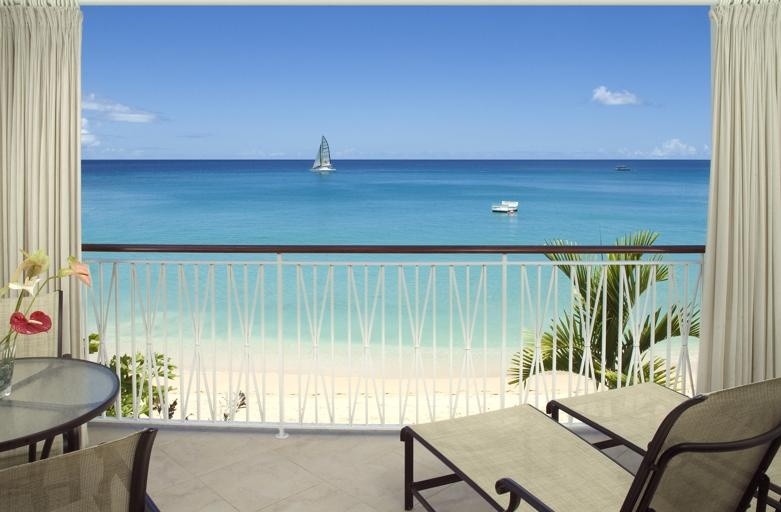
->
[0,242,89,368]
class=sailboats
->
[308,136,336,175]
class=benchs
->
[544,381,780,512]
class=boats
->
[491,200,519,213]
[615,165,630,171]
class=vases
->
[0,337,16,399]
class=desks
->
[0,355,120,509]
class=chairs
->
[0,427,157,511]
[395,377,779,512]
[0,291,62,357]
